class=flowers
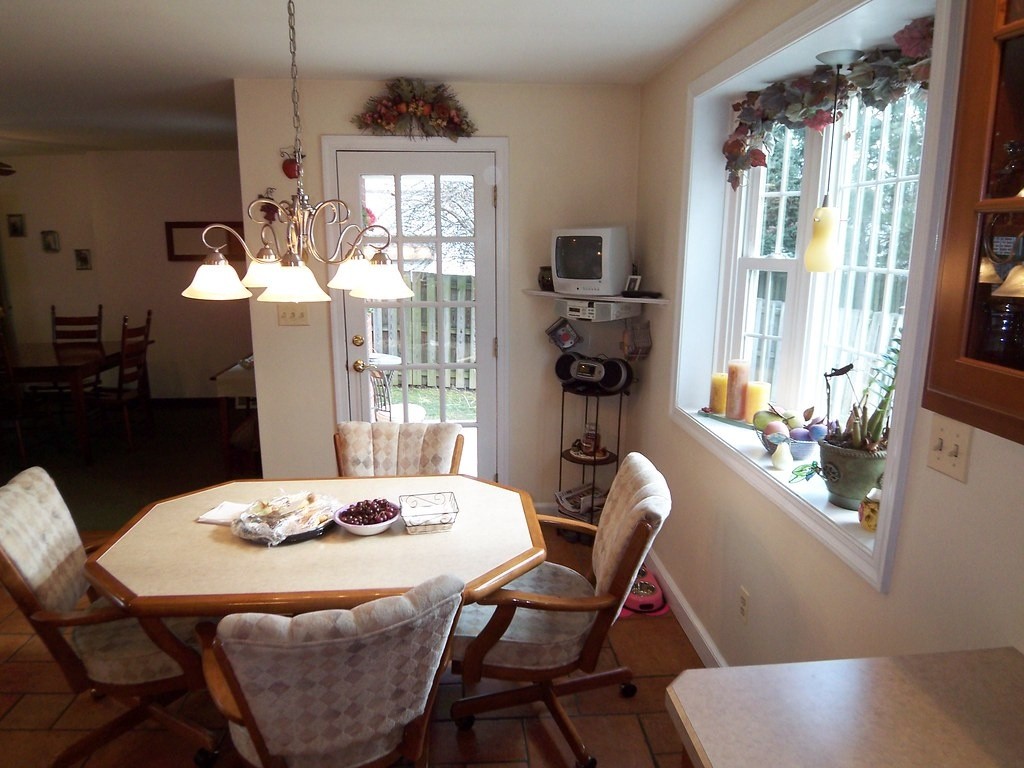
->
[353,76,478,143]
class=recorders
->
[555,352,633,393]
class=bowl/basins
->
[754,428,818,460]
[333,502,400,535]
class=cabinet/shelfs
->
[558,382,625,546]
[920,0,1024,442]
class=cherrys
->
[340,499,398,524]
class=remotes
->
[621,291,662,299]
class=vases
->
[401,115,423,135]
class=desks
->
[81,477,546,767]
[664,646,1024,768]
[9,340,155,451]
[371,354,402,406]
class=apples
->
[754,410,800,438]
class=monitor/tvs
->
[551,225,632,296]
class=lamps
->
[182,0,415,301]
[803,50,867,271]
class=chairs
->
[334,422,463,478]
[0,305,152,469]
[194,574,465,768]
[0,466,232,768]
[449,451,671,768]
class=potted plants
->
[818,328,904,511]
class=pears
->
[773,442,793,469]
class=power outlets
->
[738,585,749,623]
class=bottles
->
[544,316,580,350]
[772,442,793,469]
[858,488,882,532]
[582,423,601,454]
[538,266,554,292]
